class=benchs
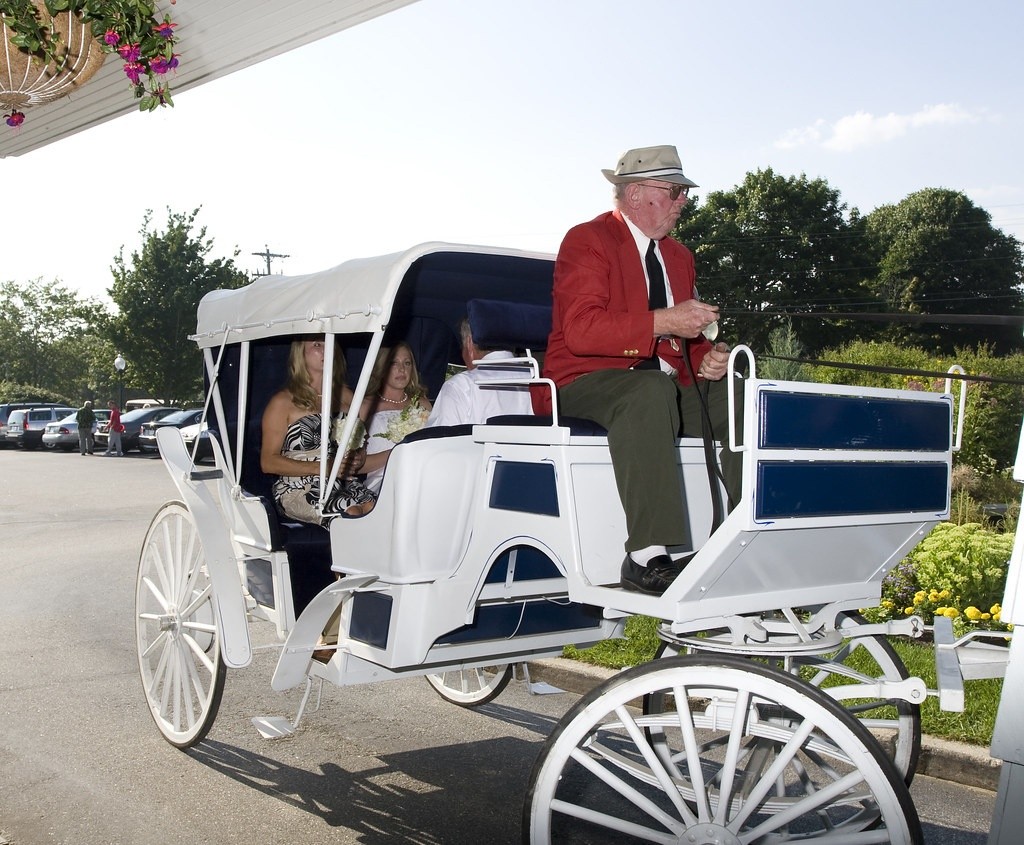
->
[465,297,728,614]
[207,399,485,636]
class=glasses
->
[637,184,689,200]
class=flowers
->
[0,1,181,113]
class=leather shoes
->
[620,552,697,597]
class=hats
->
[600,144,699,187]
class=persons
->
[104,401,123,457]
[261,336,376,661]
[76,401,96,455]
[425,317,540,428]
[357,342,432,496]
[528,146,746,596]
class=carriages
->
[130,238,1024,844]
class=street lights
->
[113,352,125,414]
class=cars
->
[0,402,212,462]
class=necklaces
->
[375,392,408,403]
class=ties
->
[632,238,667,370]
